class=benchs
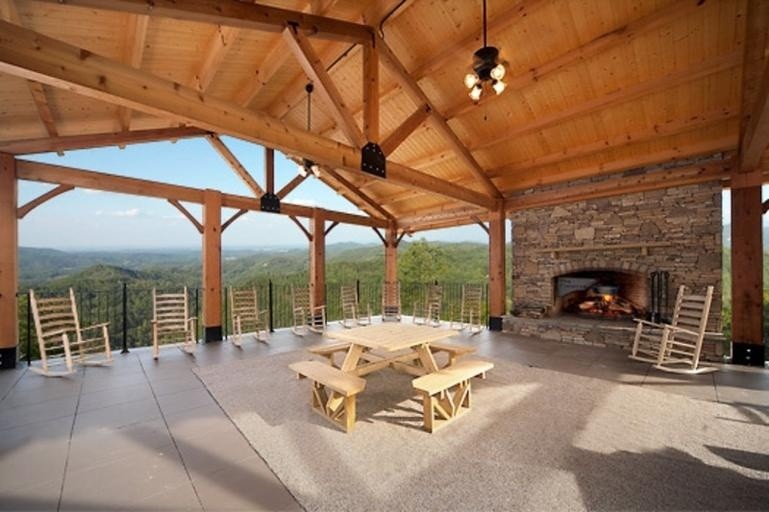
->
[288,342,494,434]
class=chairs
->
[229,285,268,346]
[628,284,720,375]
[339,285,372,328]
[290,283,327,336]
[22,287,114,376]
[412,284,443,327]
[450,285,483,335]
[381,281,402,322]
[150,287,198,359]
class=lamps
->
[463,0,508,101]
[299,82,320,179]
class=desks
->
[323,323,459,418]
